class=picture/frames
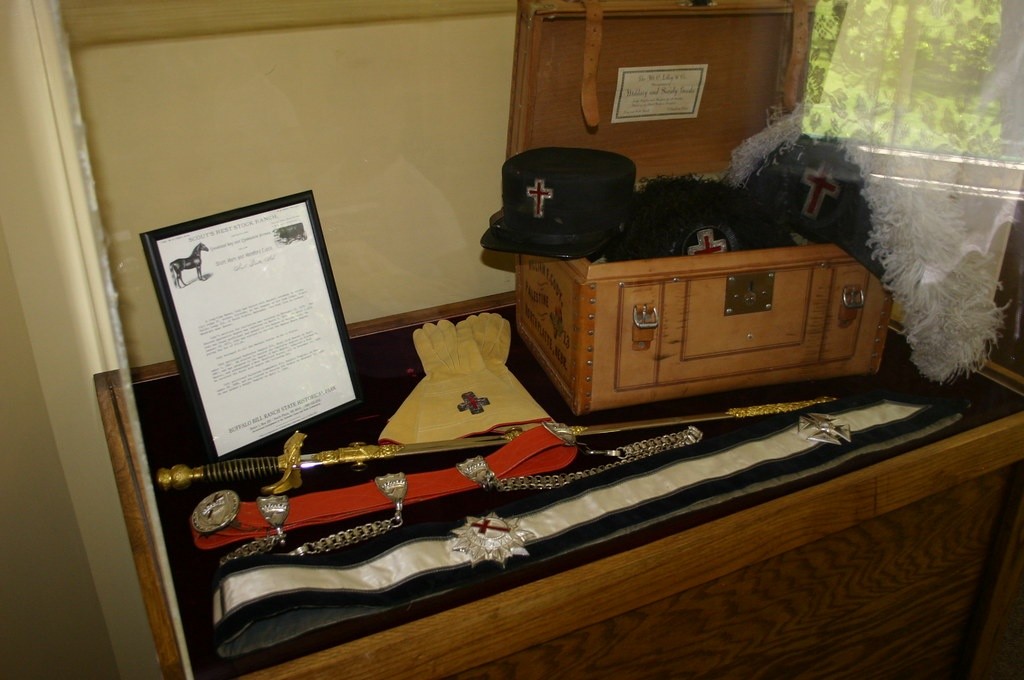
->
[140,190,364,462]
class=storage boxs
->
[504,3,892,420]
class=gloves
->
[378,313,554,443]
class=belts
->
[208,401,930,645]
[193,427,577,550]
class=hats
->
[479,148,635,259]
